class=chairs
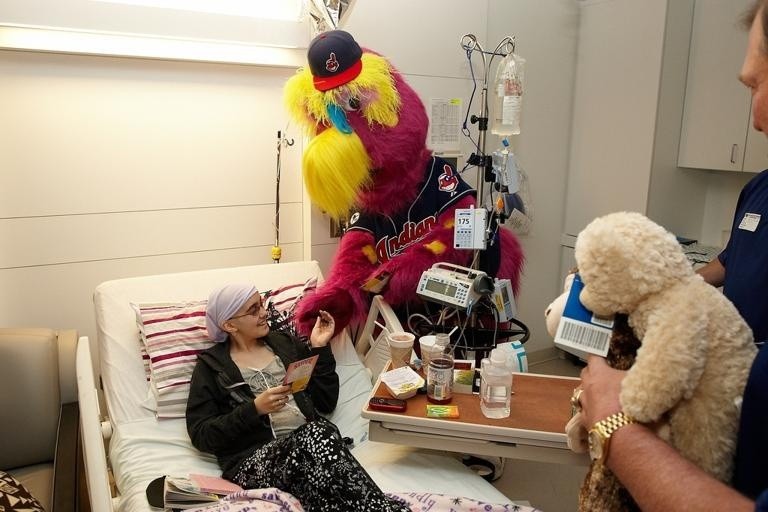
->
[0,327,63,512]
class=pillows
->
[129,273,330,424]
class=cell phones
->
[368,397,408,412]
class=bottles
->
[426,333,455,405]
[479,350,512,420]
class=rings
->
[278,400,281,405]
[571,389,582,408]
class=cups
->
[389,334,445,374]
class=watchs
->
[587,412,639,466]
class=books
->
[188,474,244,496]
[164,474,219,510]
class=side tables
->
[361,355,595,471]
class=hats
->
[308,30,362,91]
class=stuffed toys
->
[283,30,524,480]
[545,266,671,511]
[575,210,761,487]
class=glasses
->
[228,299,266,320]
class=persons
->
[186,281,396,512]
[572,0,768,511]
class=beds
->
[74,260,549,510]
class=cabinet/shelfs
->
[675,1,766,176]
